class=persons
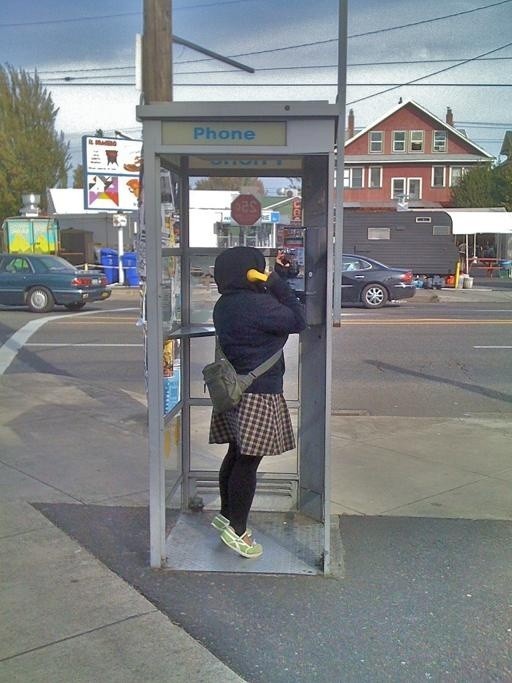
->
[274,248,291,281]
[204,247,307,558]
[456,238,496,267]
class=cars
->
[287,251,418,310]
[0,250,113,314]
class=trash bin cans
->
[100,247,139,286]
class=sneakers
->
[220,526,262,558]
[212,514,252,537]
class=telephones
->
[247,269,268,283]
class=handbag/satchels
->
[203,360,242,414]
